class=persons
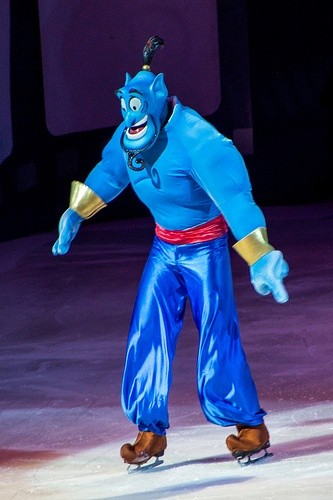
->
[51,68,288,463]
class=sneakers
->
[121,430,167,474]
[226,423,273,467]
